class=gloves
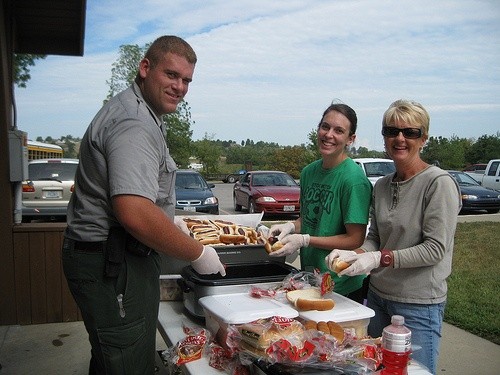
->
[191,245,226,277]
[337,251,381,277]
[267,222,295,240]
[324,249,357,270]
[269,233,310,257]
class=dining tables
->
[158,301,432,375]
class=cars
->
[233,170,301,217]
[446,164,500,215]
[221,169,248,183]
[175,168,219,215]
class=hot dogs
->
[264,237,284,253]
[183,218,267,246]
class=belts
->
[62,238,157,255]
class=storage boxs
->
[178,263,301,325]
[199,293,300,349]
[273,288,376,339]
[213,245,286,265]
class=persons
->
[267,102,374,305]
[324,98,463,375]
[61,35,227,375]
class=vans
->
[352,158,397,188]
[22,158,79,223]
[481,159,500,193]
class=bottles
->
[381,315,412,375]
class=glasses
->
[381,125,422,140]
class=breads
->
[286,288,334,310]
[331,258,350,273]
[236,319,344,358]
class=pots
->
[178,263,301,317]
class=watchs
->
[381,250,391,268]
